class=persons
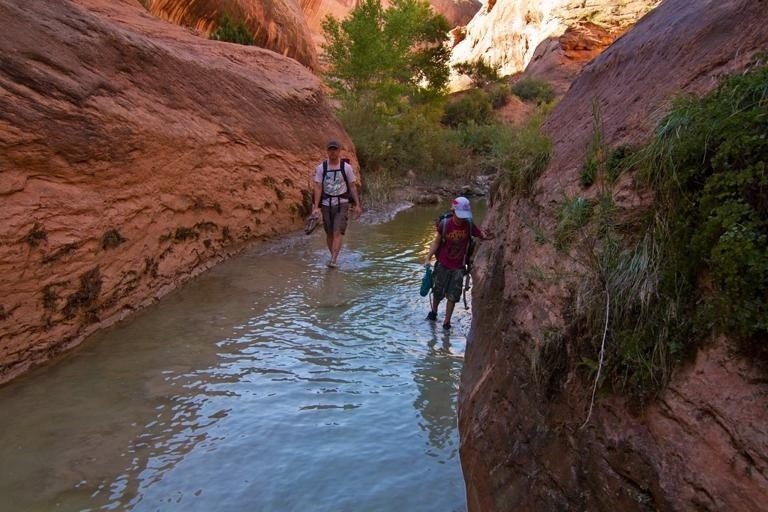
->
[418,196,481,330]
[304,139,361,269]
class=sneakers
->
[425,311,437,320]
[442,322,451,330]
[304,212,320,234]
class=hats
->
[451,196,473,219]
[327,141,339,149]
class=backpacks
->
[318,158,355,208]
[439,212,473,275]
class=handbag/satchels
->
[420,267,434,296]
